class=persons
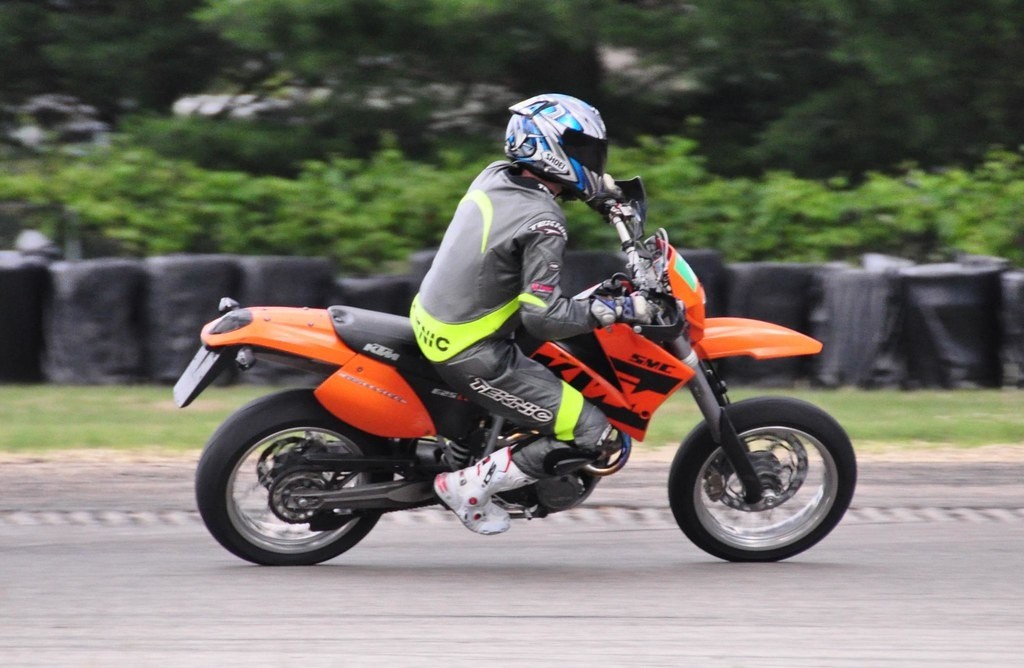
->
[410,94,659,536]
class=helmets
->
[504,93,610,198]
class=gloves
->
[589,290,656,326]
[596,172,615,196]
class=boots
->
[433,445,538,536]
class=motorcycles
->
[169,175,861,566]
